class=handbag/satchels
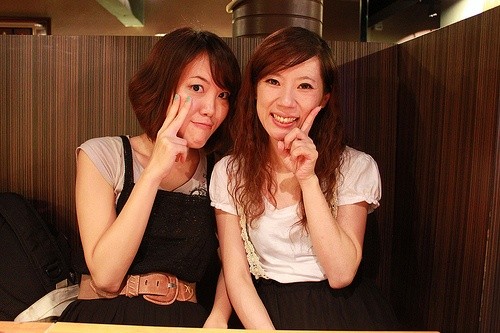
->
[14,278,80,323]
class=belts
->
[77,271,198,307]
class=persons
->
[58,26,242,329]
[208,26,382,332]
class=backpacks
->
[0,191,81,321]
